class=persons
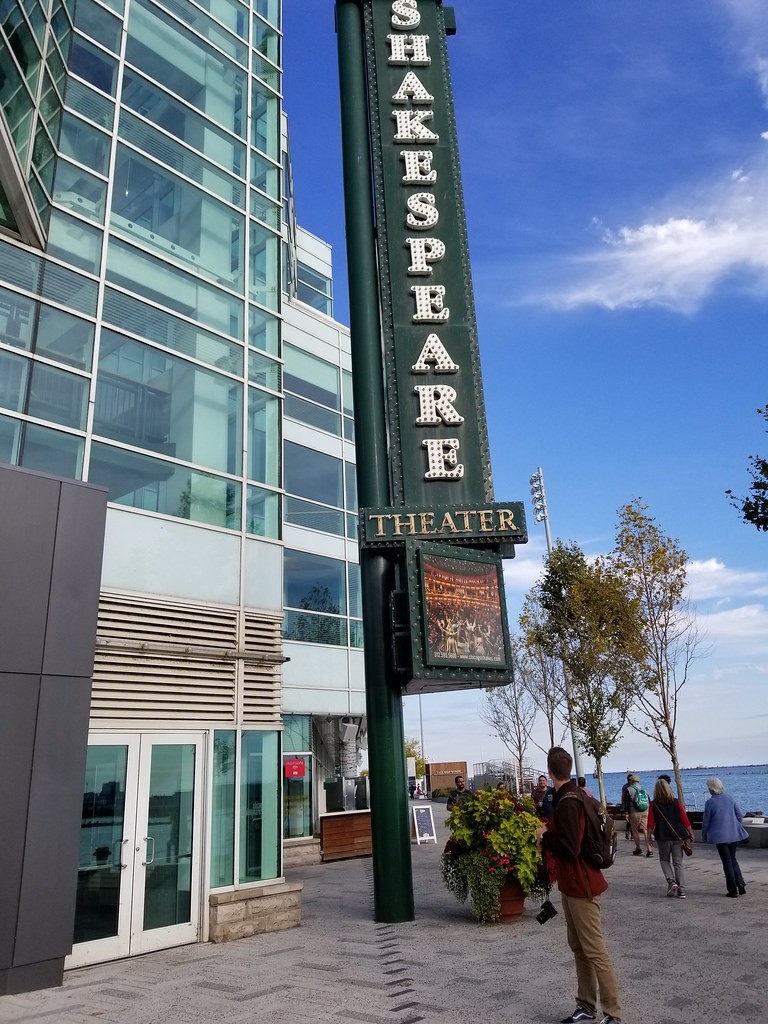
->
[497,783,513,796]
[577,777,592,797]
[447,776,471,811]
[537,748,622,1024]
[531,776,556,883]
[702,778,749,897]
[410,784,414,799]
[646,775,694,898]
[622,774,654,856]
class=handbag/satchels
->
[683,841,693,856]
[677,893,686,898]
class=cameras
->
[536,900,558,925]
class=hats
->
[629,776,640,781]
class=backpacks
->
[555,791,618,869]
[630,784,649,811]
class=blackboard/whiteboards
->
[410,804,438,847]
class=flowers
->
[439,784,554,925]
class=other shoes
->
[633,848,643,855]
[738,884,746,895]
[667,882,678,897]
[725,892,737,897]
[646,852,653,857]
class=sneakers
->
[598,1016,616,1024]
[561,1006,596,1024]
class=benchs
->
[686,811,704,831]
[606,806,627,821]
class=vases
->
[499,878,526,923]
